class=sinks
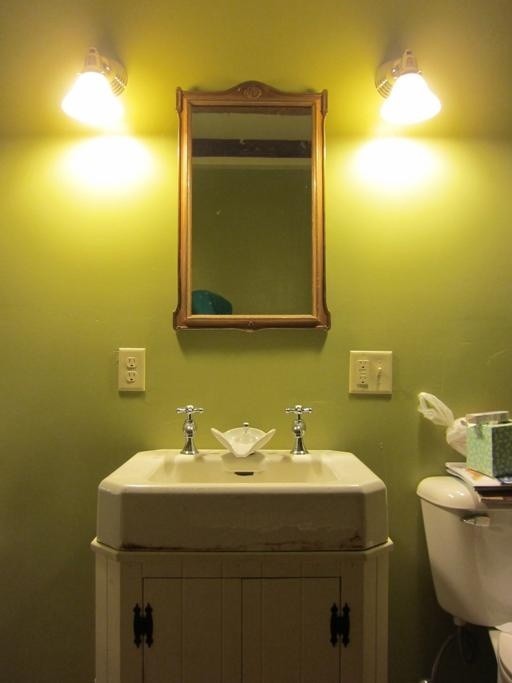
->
[98,449,387,495]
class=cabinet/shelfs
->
[90,536,394,681]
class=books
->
[444,461,512,506]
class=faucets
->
[210,417,275,459]
[176,403,205,454]
[283,405,313,454]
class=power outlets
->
[347,349,395,396]
[117,347,147,392]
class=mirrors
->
[172,81,331,333]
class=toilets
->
[417,474,512,682]
